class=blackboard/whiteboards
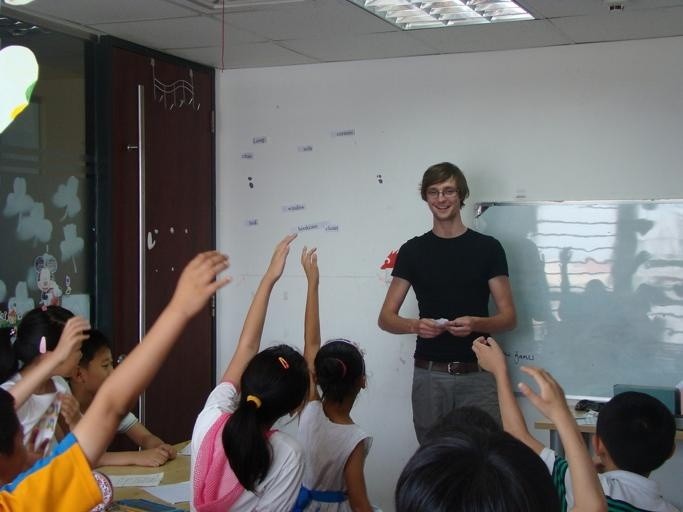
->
[473,198,683,403]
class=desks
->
[93,435,193,511]
[535,407,683,512]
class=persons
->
[0,248,234,511]
[394,362,610,512]
[189,231,312,512]
[378,160,519,451]
[470,331,682,512]
[48,328,178,470]
[273,243,380,512]
[0,305,89,472]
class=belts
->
[415,360,478,376]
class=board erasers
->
[613,384,677,417]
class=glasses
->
[426,189,459,198]
